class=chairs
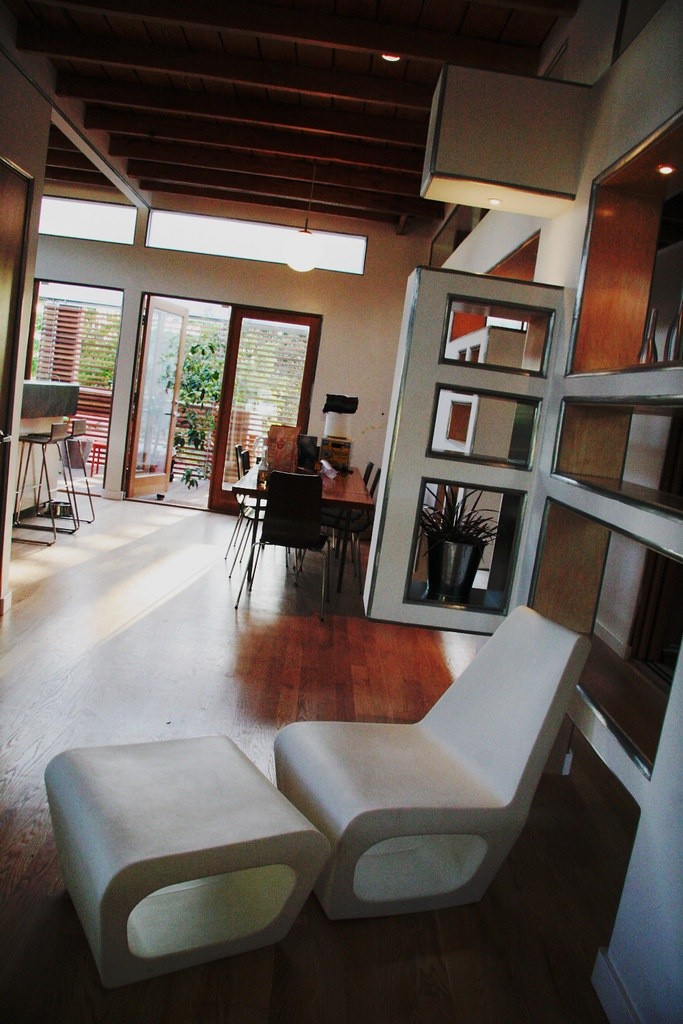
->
[273,606,591,921]
[299,462,375,563]
[235,470,330,623]
[235,444,268,510]
[298,435,318,471]
[297,467,381,594]
[225,449,291,568]
[229,494,303,587]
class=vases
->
[60,436,97,469]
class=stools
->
[12,423,77,547]
[90,439,107,477]
[45,733,330,991]
[16,418,96,531]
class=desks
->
[232,462,376,593]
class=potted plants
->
[149,331,225,491]
[416,485,504,605]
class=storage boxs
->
[319,438,355,469]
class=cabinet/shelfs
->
[362,265,576,637]
[527,109,683,809]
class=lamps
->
[284,161,324,272]
